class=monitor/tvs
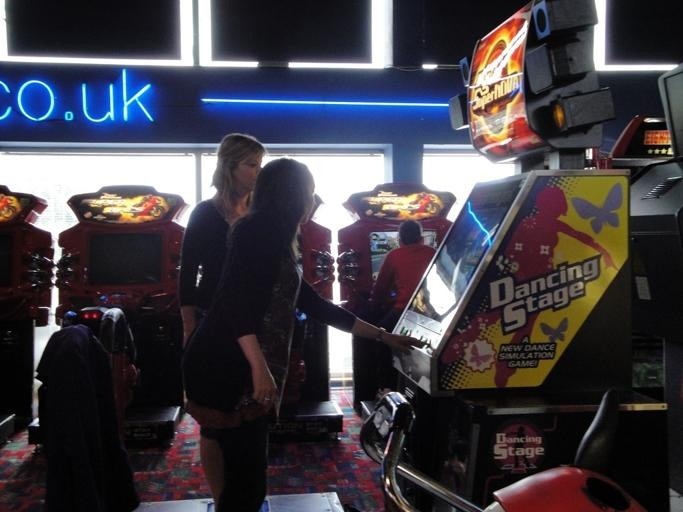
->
[367,230,438,287]
[402,176,527,334]
[87,231,165,286]
[0,231,13,290]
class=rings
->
[265,396,270,401]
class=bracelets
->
[375,326,386,341]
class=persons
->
[180,157,431,512]
[178,132,267,512]
[358,218,436,363]
